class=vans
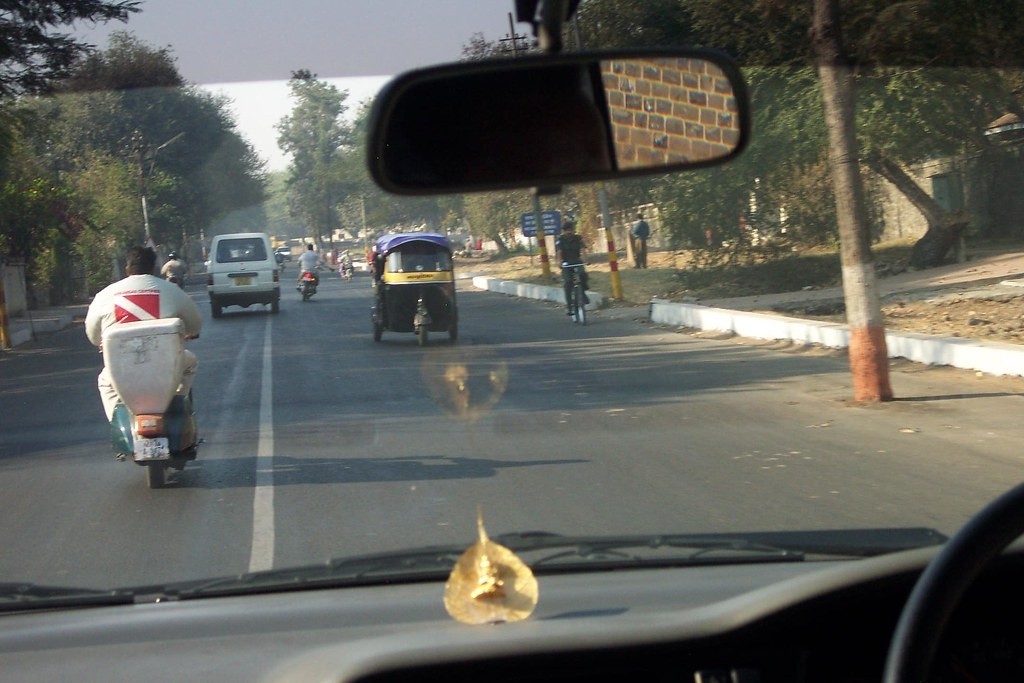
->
[207,232,285,317]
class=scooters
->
[168,271,187,290]
[341,261,355,282]
[111,333,204,489]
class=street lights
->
[138,132,186,249]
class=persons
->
[554,222,591,316]
[627,213,650,269]
[160,250,193,281]
[340,249,354,275]
[475,235,483,250]
[276,251,285,264]
[84,245,203,471]
[464,237,471,258]
[297,244,321,294]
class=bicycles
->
[562,260,587,325]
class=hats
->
[562,222,573,229]
[169,251,178,257]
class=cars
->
[275,246,292,261]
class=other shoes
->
[584,293,589,304]
[642,265,647,268]
[633,264,640,268]
[115,452,127,462]
[567,305,572,315]
[182,446,198,461]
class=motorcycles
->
[371,233,458,351]
[295,262,323,302]
